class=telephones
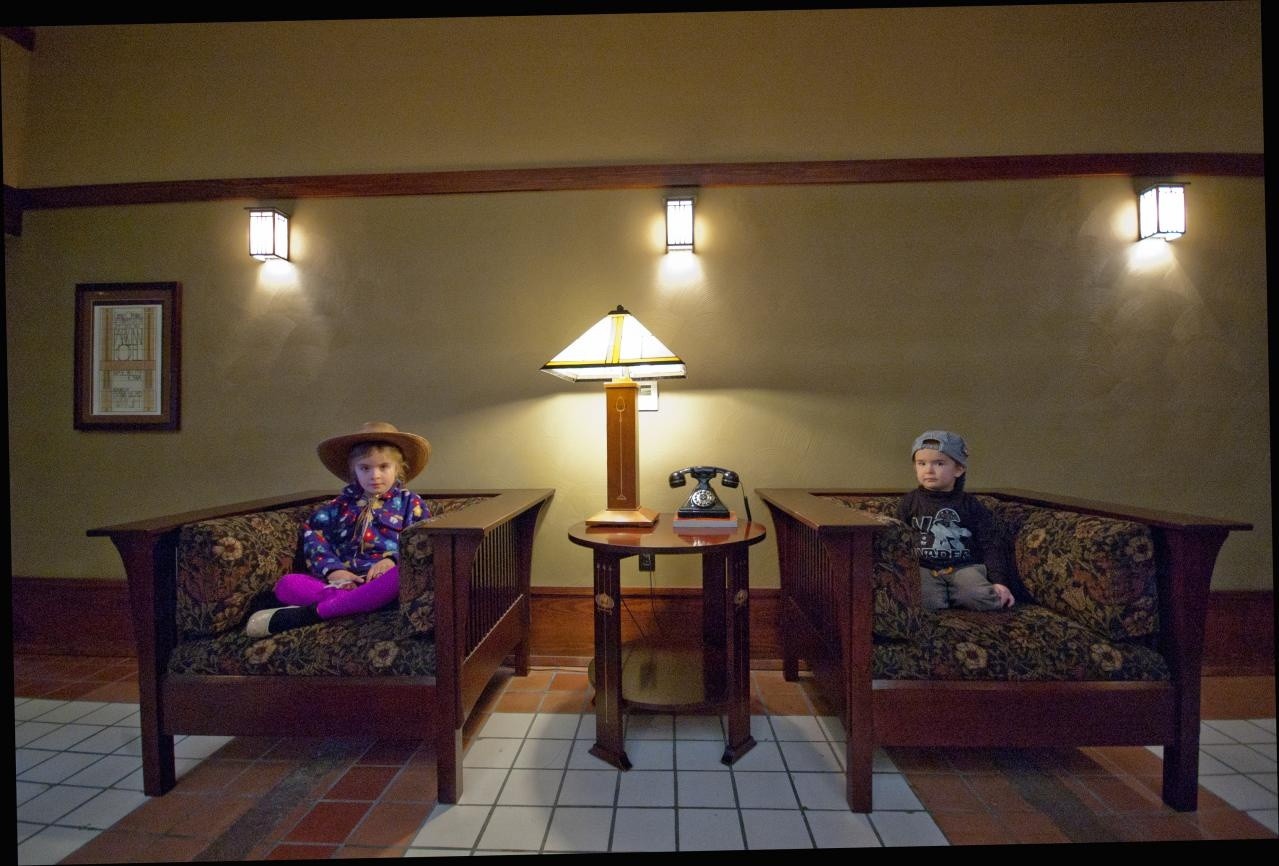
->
[670,465,740,519]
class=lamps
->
[664,196,697,255]
[244,207,290,262]
[1138,182,1192,242]
[539,303,687,528]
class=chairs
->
[756,488,1254,814]
[85,490,556,805]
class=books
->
[673,510,738,527]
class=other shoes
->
[245,605,300,638]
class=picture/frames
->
[74,281,183,432]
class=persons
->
[247,421,432,638]
[895,431,1015,612]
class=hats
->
[316,421,431,488]
[911,430,968,468]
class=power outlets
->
[639,554,654,571]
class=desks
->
[568,512,767,772]
